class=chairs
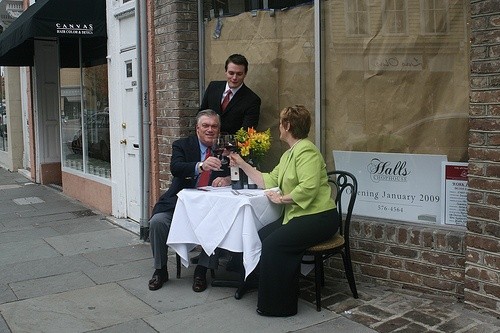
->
[298,170,358,313]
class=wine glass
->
[211,132,239,172]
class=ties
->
[221,89,231,113]
[195,148,212,189]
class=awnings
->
[67,96,85,102]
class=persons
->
[148,108,235,292]
[190,54,261,271]
[229,104,340,317]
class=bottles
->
[247,149,258,189]
[230,138,243,190]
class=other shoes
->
[256,309,261,314]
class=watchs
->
[199,162,205,173]
[280,196,284,203]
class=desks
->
[165,184,284,302]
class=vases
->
[247,149,258,189]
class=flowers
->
[233,126,274,165]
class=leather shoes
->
[193,266,206,291]
[149,268,168,290]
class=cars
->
[0,103,8,137]
[72,107,110,162]
